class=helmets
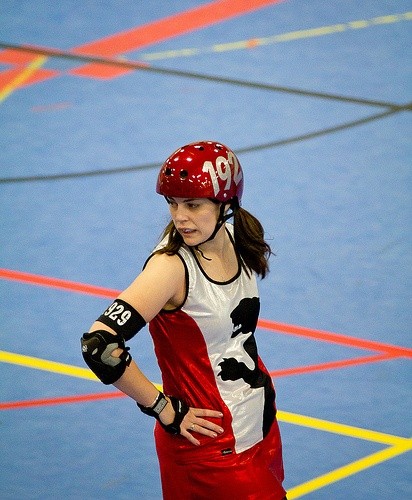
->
[155,140,243,203]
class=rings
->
[190,423,195,431]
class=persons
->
[80,140,288,500]
